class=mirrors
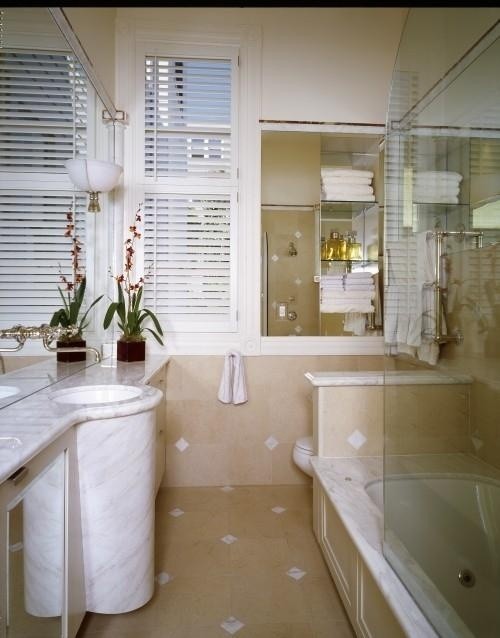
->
[7,450,68,638]
[1,8,115,410]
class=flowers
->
[104,200,164,347]
[48,202,104,343]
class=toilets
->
[293,434,317,480]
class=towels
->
[321,168,376,202]
[413,172,463,204]
[217,351,249,405]
[320,272,376,336]
[383,230,447,367]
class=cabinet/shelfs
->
[261,132,384,338]
[0,427,87,638]
[146,364,165,499]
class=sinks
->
[0,384,21,400]
[46,380,143,405]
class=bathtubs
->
[309,448,500,638]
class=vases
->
[117,341,146,362]
[57,342,86,363]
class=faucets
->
[43,338,101,364]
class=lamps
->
[87,159,124,212]
[63,158,87,212]
